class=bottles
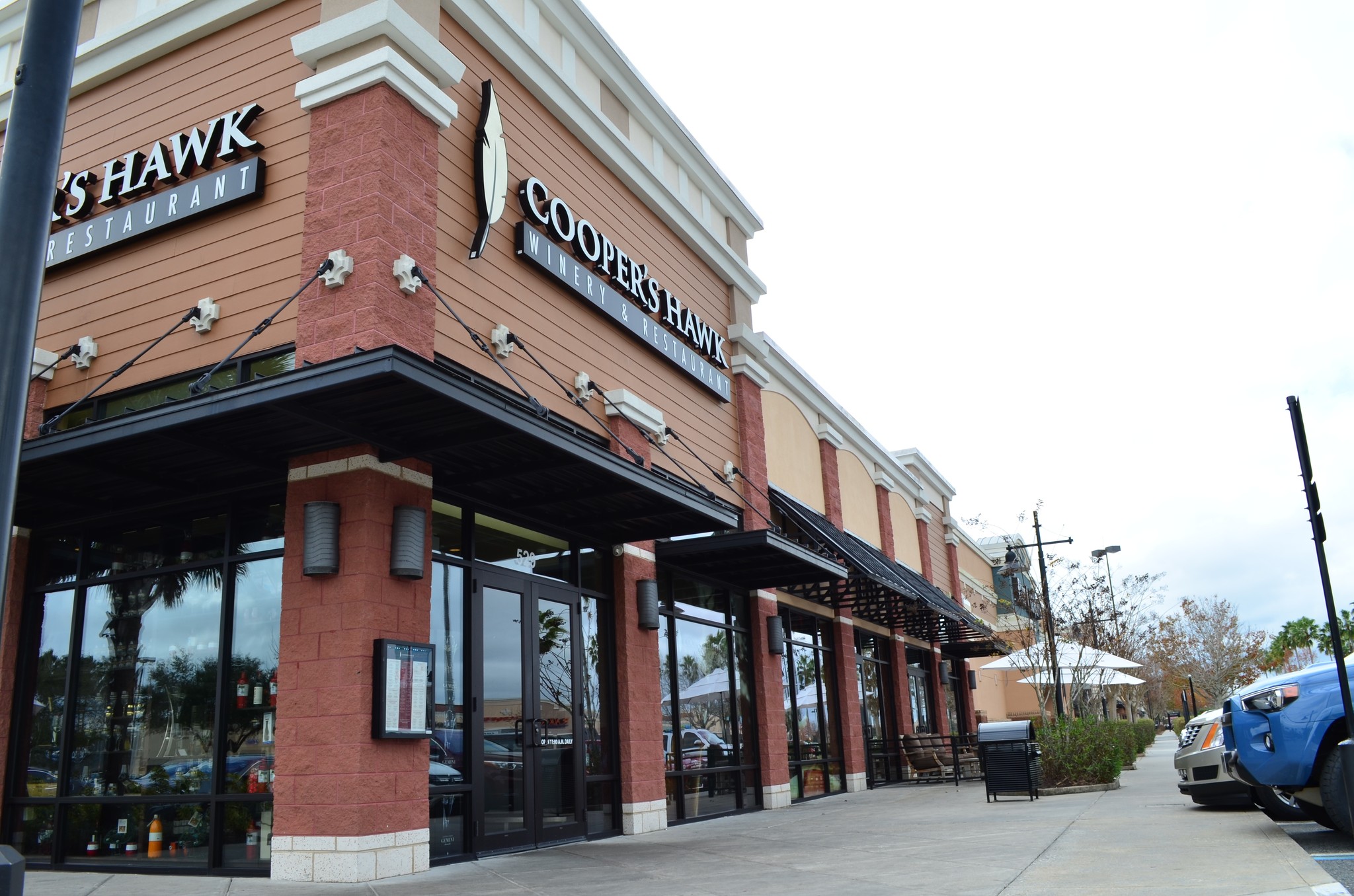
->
[147,815,162,859]
[253,671,264,707]
[237,671,248,708]
[247,818,259,859]
[270,757,275,792]
[259,756,269,793]
[270,671,278,706]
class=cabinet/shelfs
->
[35,659,156,858]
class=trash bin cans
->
[977,720,1042,802]
[542,747,576,818]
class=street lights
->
[1070,599,1116,722]
[996,510,1074,719]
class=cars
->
[22,729,743,816]
[1173,648,1354,846]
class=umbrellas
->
[1017,664,1147,687]
[659,665,792,758]
[980,633,1144,700]
[782,679,876,711]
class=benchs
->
[898,733,974,783]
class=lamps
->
[636,577,660,630]
[766,615,784,655]
[303,499,340,577]
[391,501,428,579]
[968,670,977,690]
[948,672,955,691]
[938,662,950,685]
